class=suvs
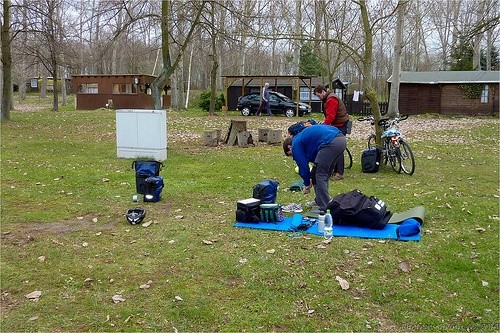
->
[236,89,312,118]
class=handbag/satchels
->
[260,203,284,223]
[235,197,261,222]
[381,120,400,139]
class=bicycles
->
[358,111,415,176]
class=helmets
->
[126,207,146,225]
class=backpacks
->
[253,179,279,204]
[361,148,381,173]
[287,119,319,134]
[143,176,165,202]
[290,179,304,191]
[325,189,394,228]
[131,159,165,195]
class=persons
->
[256,83,272,116]
[283,125,347,210]
[313,84,349,180]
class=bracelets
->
[305,186,309,190]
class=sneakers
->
[305,200,318,207]
[330,172,344,180]
[281,203,304,212]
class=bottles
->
[318,211,325,234]
[324,209,333,240]
